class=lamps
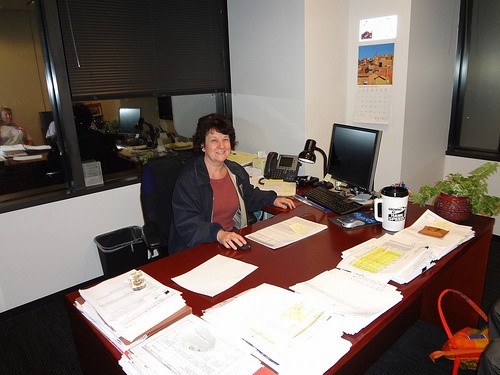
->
[298,139,327,179]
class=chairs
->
[140,152,199,260]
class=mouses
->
[342,218,365,228]
[234,243,251,249]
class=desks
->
[114,142,156,170]
[65,201,495,375]
[4,147,50,167]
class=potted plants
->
[408,162,500,223]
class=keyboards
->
[301,186,365,215]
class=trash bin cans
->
[93,225,148,279]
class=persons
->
[0,103,143,178]
[167,114,296,259]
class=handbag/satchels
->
[429,288,489,375]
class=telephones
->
[264,152,299,182]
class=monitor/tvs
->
[119,107,142,132]
[328,124,382,205]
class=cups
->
[374,186,409,231]
